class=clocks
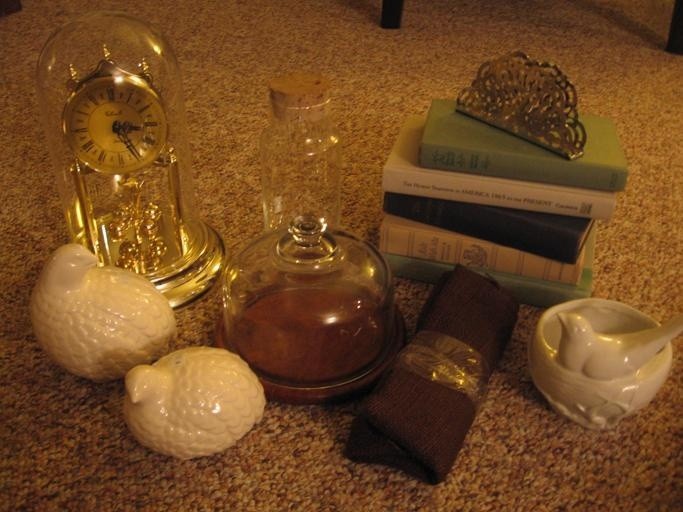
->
[36,7,225,311]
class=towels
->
[343,260,520,486]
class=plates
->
[214,280,410,400]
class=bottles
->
[254,75,345,226]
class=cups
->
[530,299,670,425]
[221,215,391,380]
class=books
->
[379,217,589,287]
[419,99,629,194]
[382,191,594,264]
[380,111,619,227]
[374,225,596,308]
